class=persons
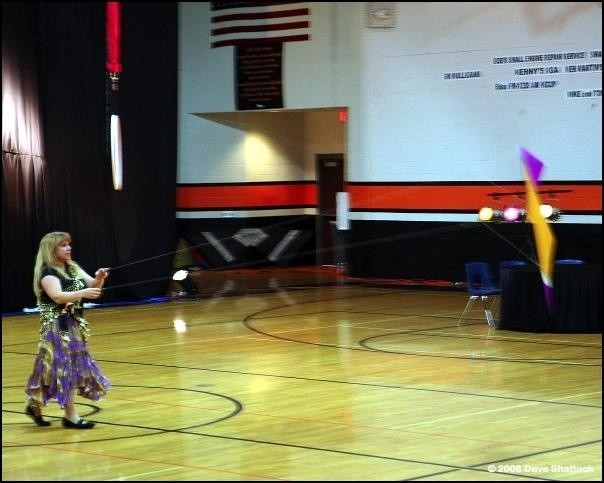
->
[26,231,111,429]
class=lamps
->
[172,268,199,295]
[476,187,580,224]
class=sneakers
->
[62,418,94,428]
[28,406,50,426]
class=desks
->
[495,263,604,336]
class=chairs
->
[501,259,531,267]
[556,259,584,264]
[456,261,501,328]
